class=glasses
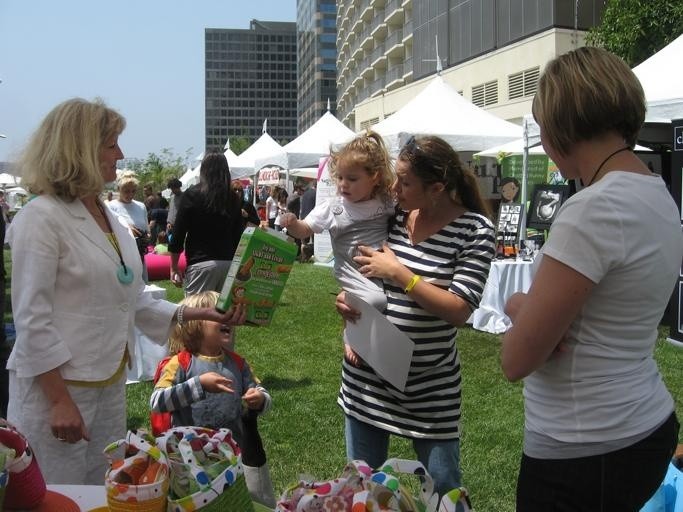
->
[403,135,445,174]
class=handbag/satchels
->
[158,425,255,512]
[0,419,48,512]
[102,429,173,512]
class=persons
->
[168,153,259,297]
[6,99,248,486]
[232,178,316,264]
[499,177,521,205]
[280,131,400,369]
[149,292,275,511]
[335,135,496,512]
[104,168,185,285]
[537,191,547,219]
[501,47,683,511]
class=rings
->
[58,438,65,441]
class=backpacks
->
[149,344,245,437]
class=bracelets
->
[404,275,420,293]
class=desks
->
[464,250,541,335]
[44,484,279,512]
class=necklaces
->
[585,146,630,186]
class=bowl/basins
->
[519,254,534,261]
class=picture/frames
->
[526,183,571,229]
